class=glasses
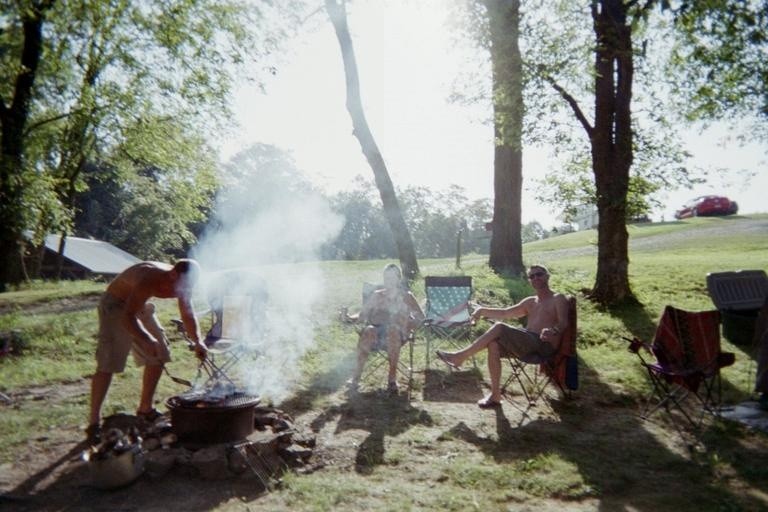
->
[529,271,546,280]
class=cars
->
[672,194,741,219]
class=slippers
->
[436,350,462,371]
[88,407,166,443]
[478,393,502,408]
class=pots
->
[163,390,261,452]
[80,440,147,492]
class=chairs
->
[624,308,738,430]
[354,274,482,378]
[169,268,268,387]
[502,295,578,411]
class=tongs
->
[199,353,218,382]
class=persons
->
[335,263,427,392]
[87,257,211,446]
[435,265,570,411]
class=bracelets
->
[552,326,559,336]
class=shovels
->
[130,340,191,387]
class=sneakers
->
[345,376,399,394]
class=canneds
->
[341,306,347,321]
